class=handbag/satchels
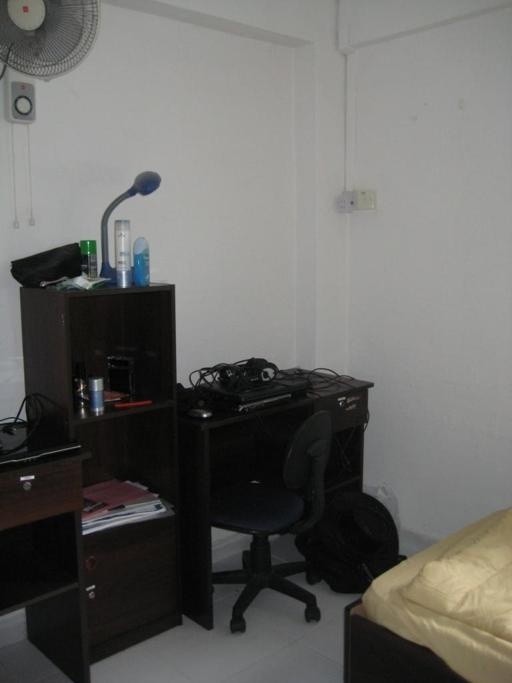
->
[10,243,82,287]
[294,486,404,584]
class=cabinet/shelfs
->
[315,390,368,520]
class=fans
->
[1,0,99,230]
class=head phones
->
[240,359,278,389]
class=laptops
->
[198,379,310,413]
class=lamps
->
[99,171,163,286]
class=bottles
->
[132,237,151,288]
[114,219,132,290]
[87,376,105,416]
[80,239,98,282]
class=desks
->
[179,367,376,629]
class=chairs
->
[212,410,335,626]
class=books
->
[103,388,130,402]
[111,399,153,407]
[80,476,168,539]
